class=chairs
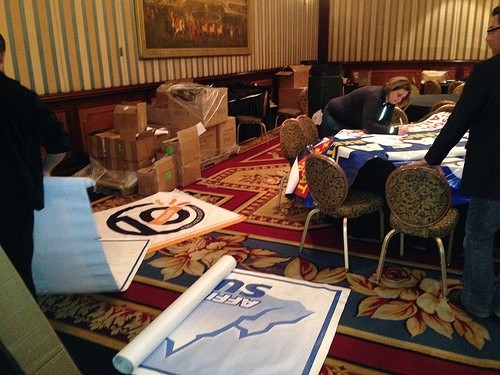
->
[237,90,268,145]
[375,164,458,297]
[299,154,384,271]
[392,80,465,124]
[277,115,318,207]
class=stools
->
[275,87,308,128]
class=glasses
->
[486,26,500,33]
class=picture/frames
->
[134,0,254,59]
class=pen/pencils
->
[399,118,403,125]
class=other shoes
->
[491,310,500,321]
[447,288,491,321]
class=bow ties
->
[383,102,391,107]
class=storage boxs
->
[86,78,237,196]
[275,65,311,115]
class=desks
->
[328,124,470,238]
[404,94,461,123]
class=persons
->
[0,36,71,301]
[323,77,411,140]
[406,7,500,318]
